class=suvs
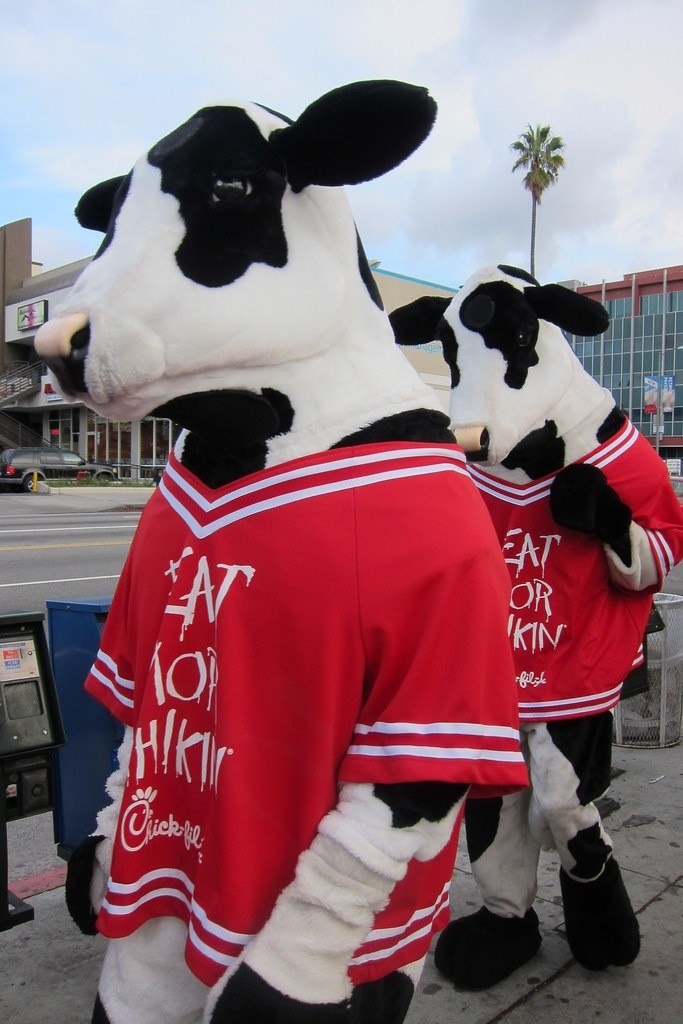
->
[0,448,117,492]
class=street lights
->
[656,345,683,456]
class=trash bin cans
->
[46,599,126,862]
[609,592,683,750]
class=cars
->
[668,475,683,510]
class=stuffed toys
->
[389,264,683,988]
[35,80,530,1024]
